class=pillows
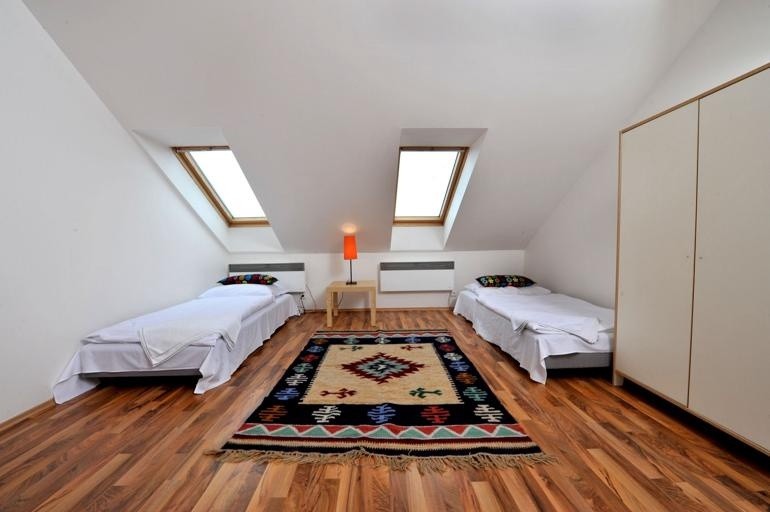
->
[197,273,283,295]
[464,274,551,297]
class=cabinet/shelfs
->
[609,63,769,456]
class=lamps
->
[343,234,360,285]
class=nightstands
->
[326,279,378,327]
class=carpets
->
[206,328,560,476]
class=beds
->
[458,289,615,369]
[78,293,294,382]
